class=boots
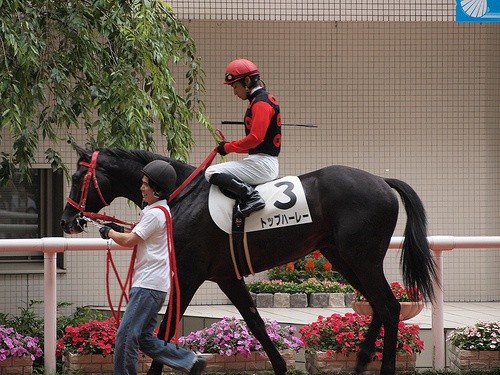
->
[208,173,265,218]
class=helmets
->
[142,159,177,195]
[224,57,261,85]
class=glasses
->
[225,73,234,82]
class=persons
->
[205,59,281,218]
[99,160,207,375]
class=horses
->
[60,142,448,375]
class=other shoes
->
[188,355,207,375]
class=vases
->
[0,292,500,375]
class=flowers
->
[56,317,177,358]
[0,324,45,362]
[298,313,426,361]
[243,250,426,301]
[179,316,305,359]
[450,320,500,350]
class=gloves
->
[103,223,125,233]
[99,226,111,239]
[217,141,230,156]
